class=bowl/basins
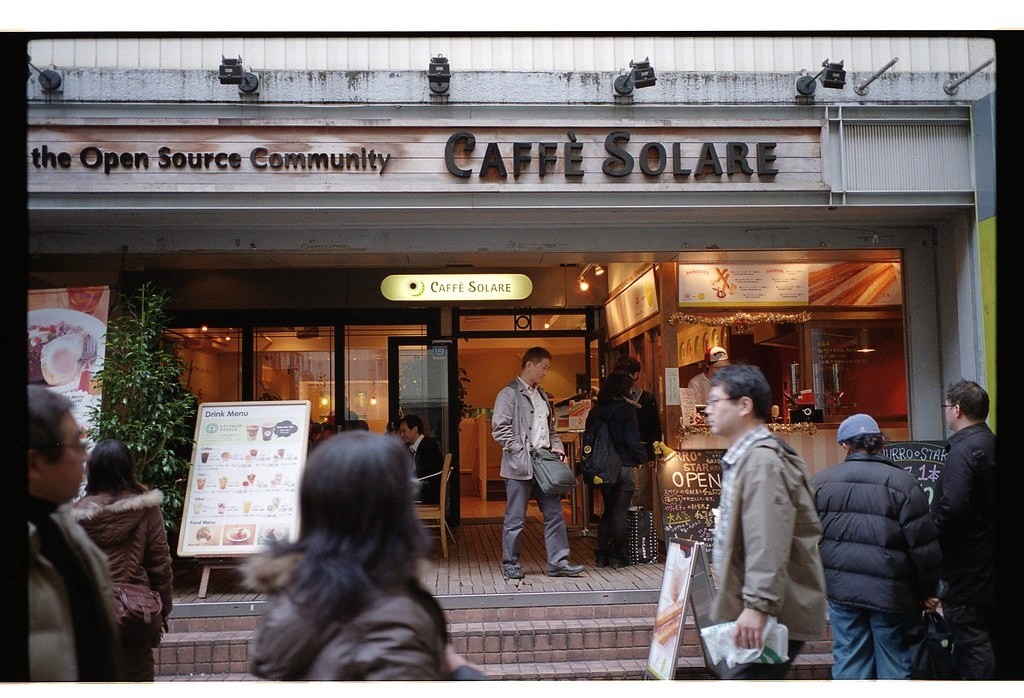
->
[79,439,88,445]
[227,528,251,541]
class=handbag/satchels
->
[111,581,164,636]
[628,488,660,565]
[925,607,996,680]
[903,606,955,680]
[532,449,578,496]
[700,615,789,668]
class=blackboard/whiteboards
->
[812,333,858,389]
[881,440,951,511]
[655,449,727,563]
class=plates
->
[263,527,285,541]
[557,413,568,419]
[194,525,213,544]
[28,308,107,393]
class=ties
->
[411,448,415,456]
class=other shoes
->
[597,556,609,567]
[610,557,625,569]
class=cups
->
[242,501,252,515]
[247,474,256,484]
[261,426,273,441]
[275,473,281,484]
[278,449,284,458]
[193,502,203,513]
[220,451,229,461]
[201,452,209,462]
[197,478,206,489]
[245,450,257,461]
[219,478,228,489]
[217,503,225,515]
[246,424,258,439]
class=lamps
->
[216,54,260,94]
[795,58,847,97]
[612,56,657,96]
[427,53,451,96]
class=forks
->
[78,334,97,392]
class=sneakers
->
[504,568,525,580]
[549,563,585,576]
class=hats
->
[836,413,880,443]
[704,346,728,362]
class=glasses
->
[704,396,742,411]
[941,404,966,413]
[60,432,89,455]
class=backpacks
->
[959,433,998,530]
[579,399,622,487]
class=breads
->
[653,600,684,645]
[570,407,585,416]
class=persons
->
[935,380,1003,680]
[687,346,730,405]
[490,347,586,580]
[71,438,174,682]
[806,413,939,680]
[584,370,646,570]
[242,430,488,681]
[617,358,661,506]
[307,415,444,506]
[703,365,827,681]
[24,384,126,682]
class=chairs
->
[413,452,452,558]
